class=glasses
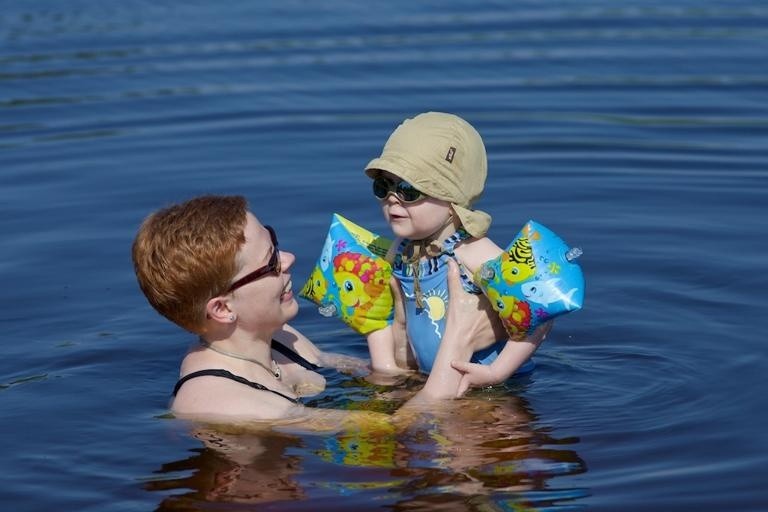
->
[369,174,430,205]
[203,224,282,321]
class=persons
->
[380,383,546,511]
[363,110,555,403]
[129,193,506,438]
[152,422,317,512]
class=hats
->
[361,108,493,241]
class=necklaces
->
[199,338,281,381]
